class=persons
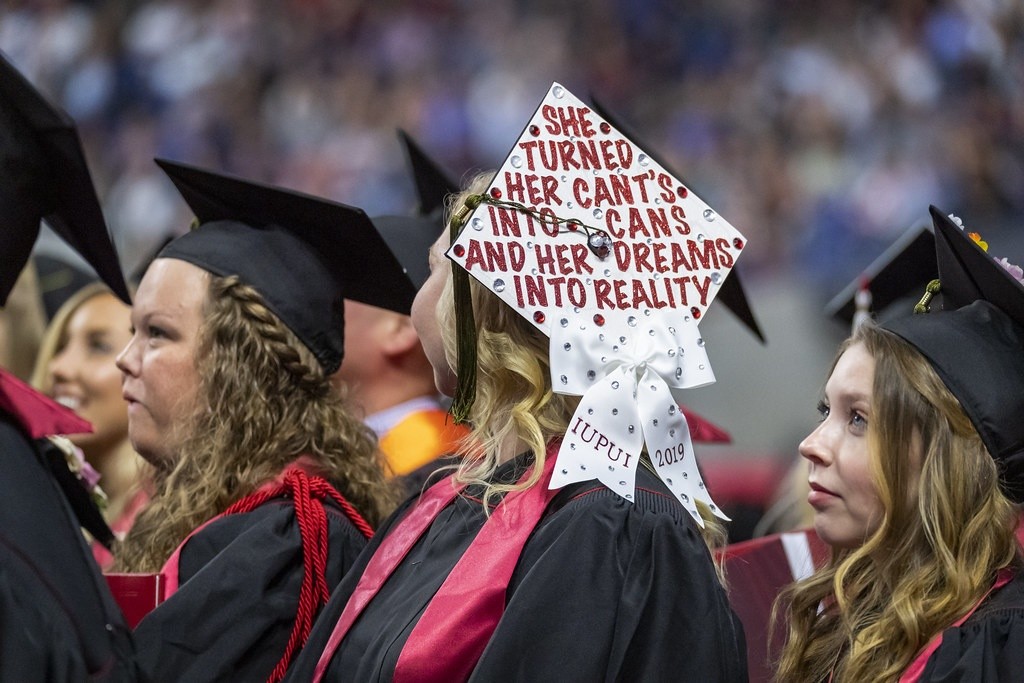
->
[118,158,418,683]
[289,78,766,683]
[333,126,480,480]
[0,52,136,683]
[767,204,1024,682]
[33,278,159,561]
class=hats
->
[154,157,419,378]
[826,225,942,330]
[0,52,133,311]
[589,90,766,345]
[879,204,1024,502]
[444,80,750,528]
